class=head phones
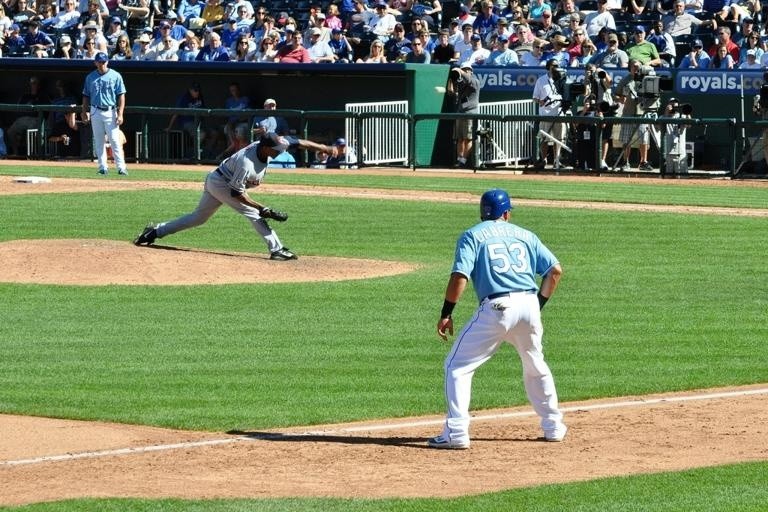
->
[546,60,550,70]
[628,58,643,72]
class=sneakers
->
[639,161,653,169]
[428,436,469,450]
[601,160,607,169]
[99,168,127,175]
[271,247,297,260]
[546,425,566,442]
[135,228,156,245]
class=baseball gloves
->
[259,207,288,221]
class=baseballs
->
[434,86,446,93]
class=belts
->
[92,105,114,111]
[488,290,535,299]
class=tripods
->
[612,97,660,172]
[524,96,572,171]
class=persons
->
[1,0,768,72]
[532,58,692,176]
[133,131,341,260]
[426,188,566,446]
[451,63,480,167]
[1,52,346,175]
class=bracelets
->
[538,291,548,313]
[442,298,456,319]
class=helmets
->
[480,190,513,220]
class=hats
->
[498,19,507,26]
[471,34,480,40]
[261,133,288,151]
[606,34,618,44]
[746,49,756,55]
[111,17,120,23]
[310,13,342,35]
[337,138,345,146]
[264,99,276,107]
[633,26,645,34]
[60,36,72,45]
[84,21,99,31]
[497,34,508,42]
[95,53,109,62]
[692,39,703,48]
[550,35,569,45]
[134,21,171,43]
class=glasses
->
[413,43,420,46]
[258,12,265,15]
[751,37,758,39]
[240,42,247,44]
